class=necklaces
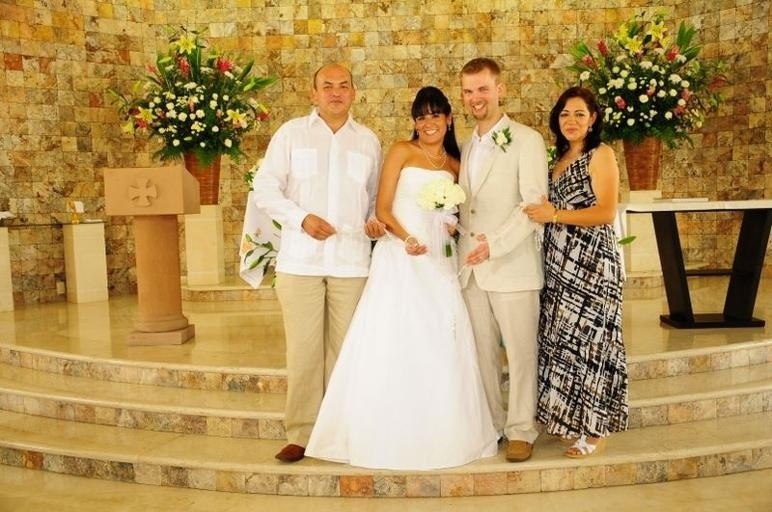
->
[418,141,448,170]
[560,153,582,165]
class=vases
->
[622,136,661,190]
[184,150,222,205]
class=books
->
[654,196,710,202]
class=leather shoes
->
[275,444,305,462]
[506,440,532,461]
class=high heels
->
[560,431,608,458]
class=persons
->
[522,87,628,459]
[253,63,382,459]
[304,88,499,471]
[454,57,549,463]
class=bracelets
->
[405,236,415,243]
[553,209,558,225]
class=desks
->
[625,200,772,329]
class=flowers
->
[565,6,732,151]
[546,143,557,178]
[240,155,281,288]
[106,16,279,169]
[490,125,513,154]
[415,174,466,258]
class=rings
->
[480,257,482,259]
